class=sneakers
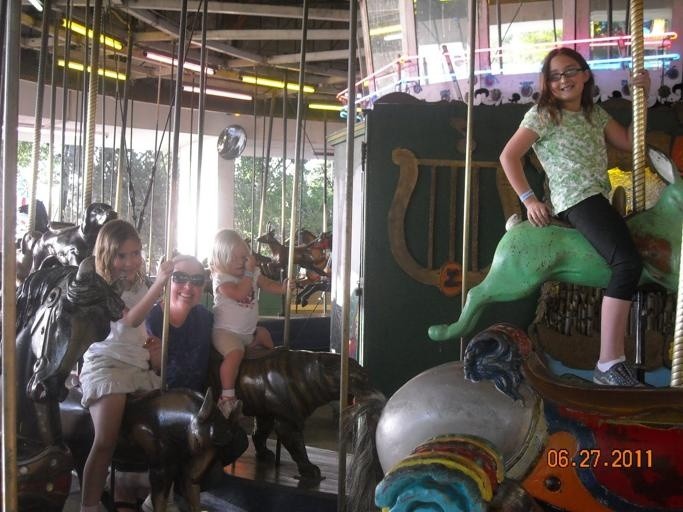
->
[142,493,178,511]
[174,474,207,512]
[218,397,243,421]
[592,361,645,388]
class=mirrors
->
[216,125,248,160]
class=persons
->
[210,230,294,420]
[79,220,174,512]
[499,50,650,389]
[145,256,248,487]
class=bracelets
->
[520,188,534,201]
[242,268,254,280]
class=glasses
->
[173,272,205,287]
[549,68,584,81]
